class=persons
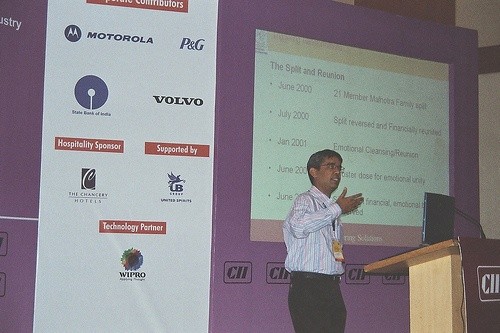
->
[282,149,366,333]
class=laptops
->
[380,192,456,263]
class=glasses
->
[318,163,345,171]
